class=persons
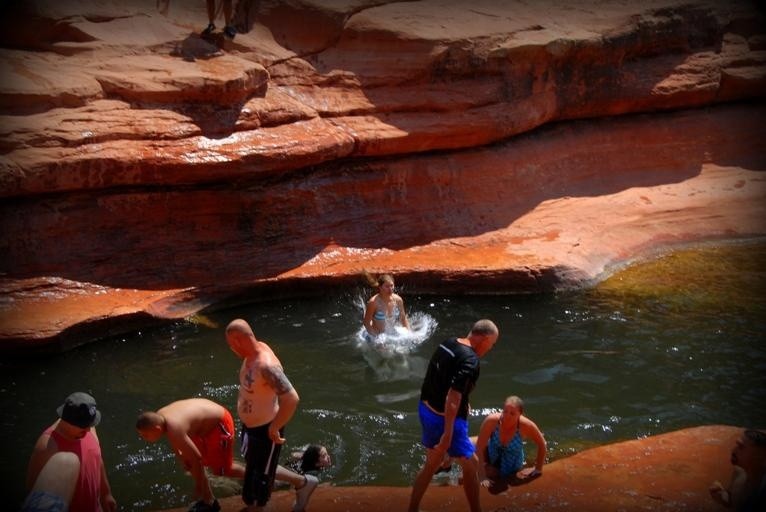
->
[707,429,766,511]
[135,395,246,511]
[474,394,545,488]
[363,274,408,339]
[406,317,499,511]
[286,442,333,480]
[224,317,320,511]
[23,390,120,511]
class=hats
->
[54,391,103,429]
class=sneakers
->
[292,472,320,510]
[186,495,221,512]
